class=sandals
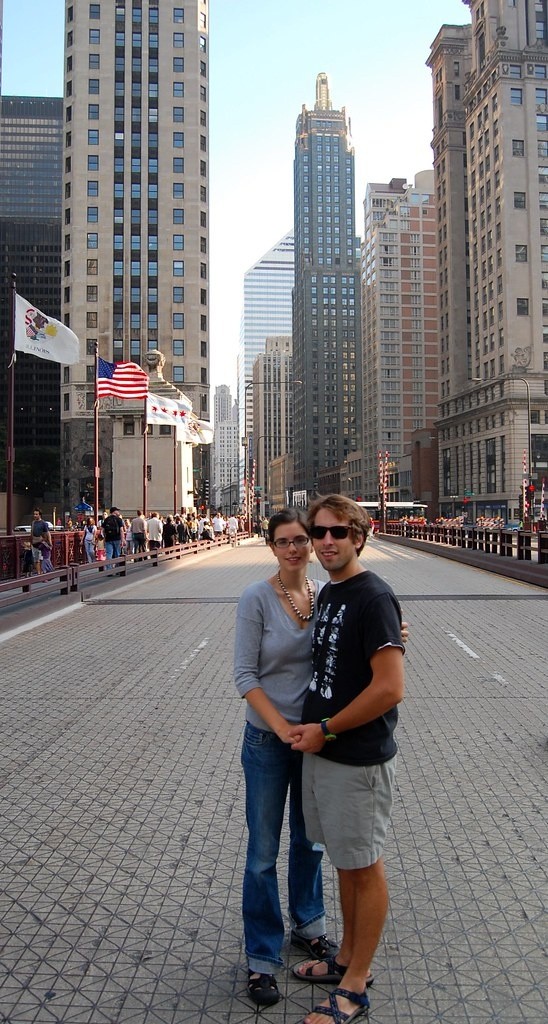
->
[291,929,339,959]
[294,955,374,987]
[303,986,370,1024]
[247,969,279,1005]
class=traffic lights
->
[525,486,531,509]
[518,493,524,526]
[203,478,210,500]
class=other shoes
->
[43,579,47,583]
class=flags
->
[14,292,80,364]
[146,392,192,426]
[177,419,214,444]
[97,356,149,400]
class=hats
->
[110,507,120,512]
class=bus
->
[354,501,428,534]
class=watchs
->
[321,718,336,742]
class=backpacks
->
[105,516,119,534]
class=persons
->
[232,508,409,1005]
[22,507,270,583]
[287,494,405,1024]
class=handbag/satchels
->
[33,536,45,544]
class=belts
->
[86,539,92,541]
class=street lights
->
[244,381,302,520]
[256,435,295,537]
[471,377,532,530]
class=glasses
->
[311,526,351,539]
[271,535,309,548]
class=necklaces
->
[276,570,314,621]
